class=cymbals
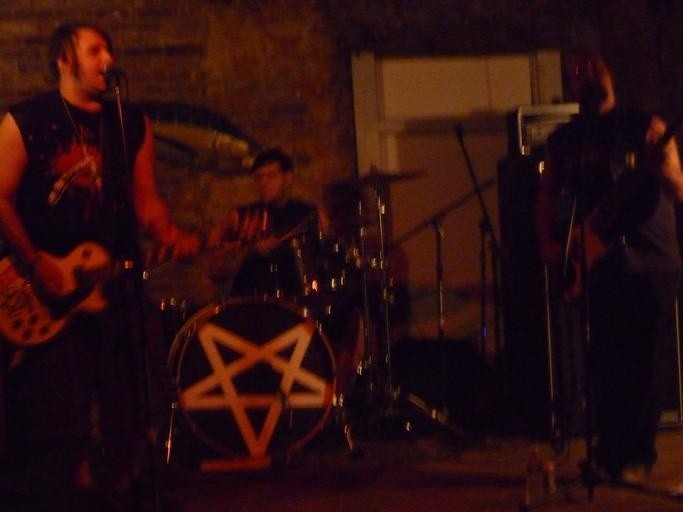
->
[140,268,217,313]
[327,172,422,189]
[336,301,366,395]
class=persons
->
[215,145,324,297]
[534,40,681,487]
[0,20,203,511]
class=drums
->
[167,292,340,466]
[256,233,363,326]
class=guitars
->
[531,114,683,304]
[0,230,248,350]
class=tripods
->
[518,193,683,512]
[354,184,482,448]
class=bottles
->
[159,296,188,326]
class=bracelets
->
[23,254,44,275]
